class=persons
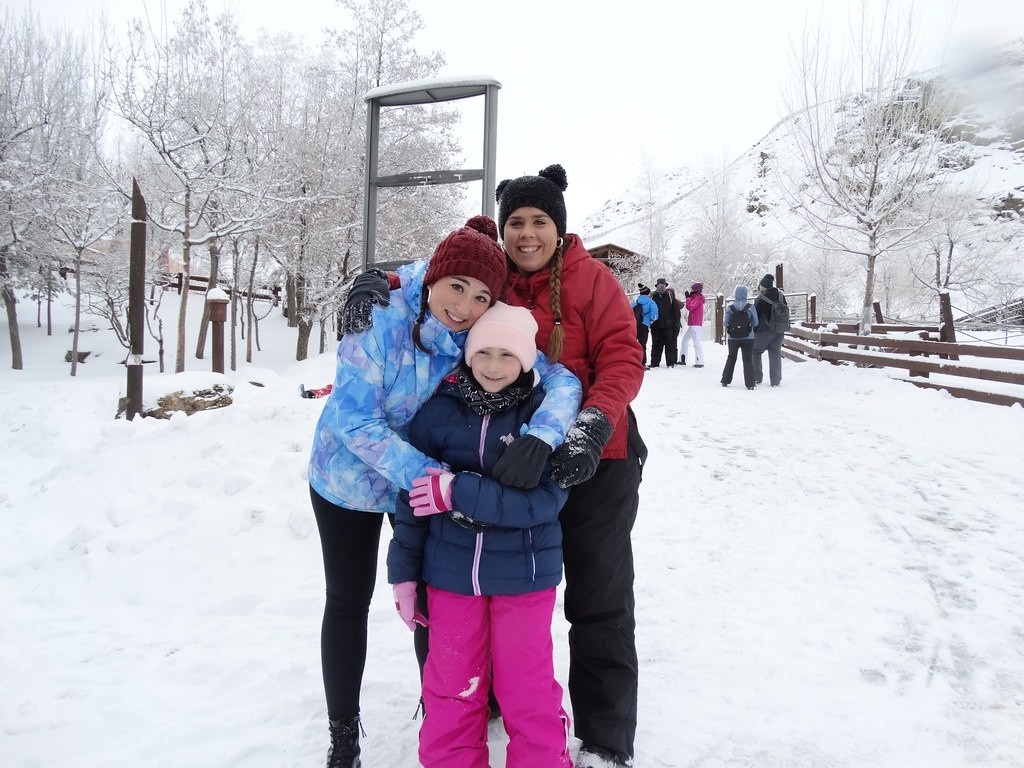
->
[720,285,760,390]
[385,300,574,768]
[343,163,649,767]
[308,215,583,768]
[676,283,705,368]
[631,283,658,370]
[752,274,789,387]
[646,278,677,368]
[667,287,684,334]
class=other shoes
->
[575,741,634,768]
[642,354,782,390]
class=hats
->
[655,278,669,287]
[691,281,705,293]
[423,215,508,304]
[759,273,774,288]
[637,283,651,295]
[494,164,568,238]
[465,301,539,373]
[666,287,675,293]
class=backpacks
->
[727,303,753,337]
[757,287,790,334]
[632,299,651,326]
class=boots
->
[326,715,367,768]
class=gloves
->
[393,581,429,632]
[408,466,457,517]
[447,510,493,534]
[550,406,613,489]
[685,290,690,298]
[337,267,391,334]
[490,434,551,489]
